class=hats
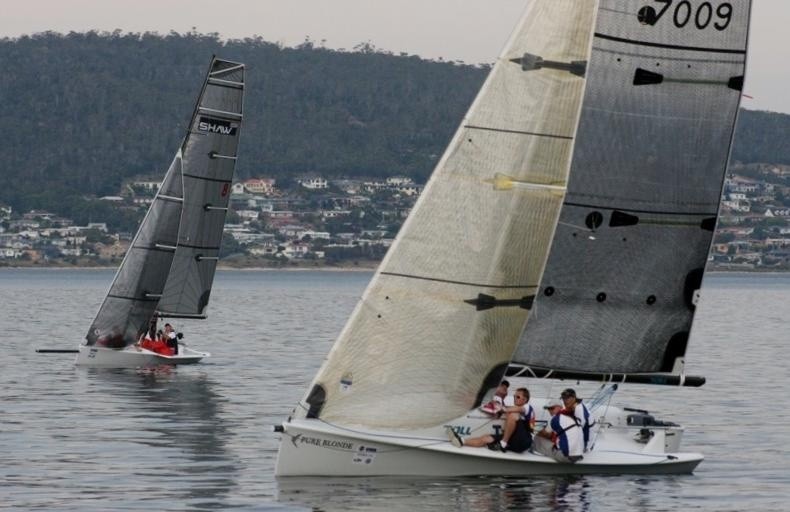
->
[543,400,562,409]
[561,389,575,399]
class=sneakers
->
[487,440,507,452]
[449,429,462,448]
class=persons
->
[531,398,585,464]
[446,387,535,453]
[482,379,509,417]
[108,320,178,356]
[559,388,595,451]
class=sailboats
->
[76,53,245,367]
[275,1,755,480]
